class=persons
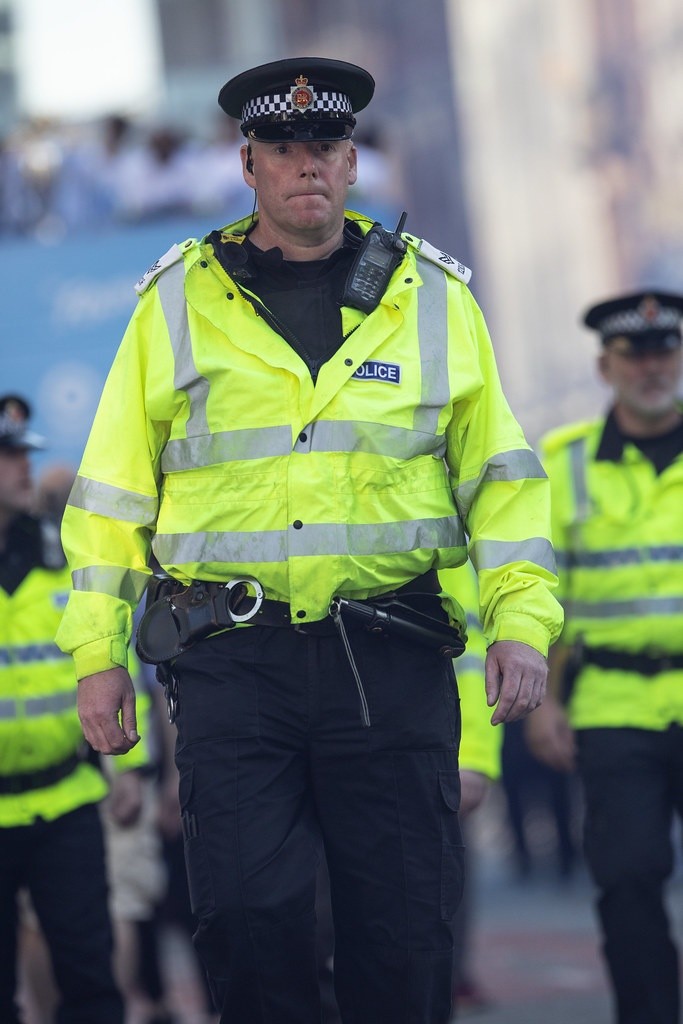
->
[1,386,156,1024]
[482,287,682,1023]
[409,493,523,1010]
[58,55,564,1023]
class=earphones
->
[245,159,254,175]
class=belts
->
[178,569,442,637]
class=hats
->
[0,397,47,450]
[585,292,682,355]
[218,57,376,143]
